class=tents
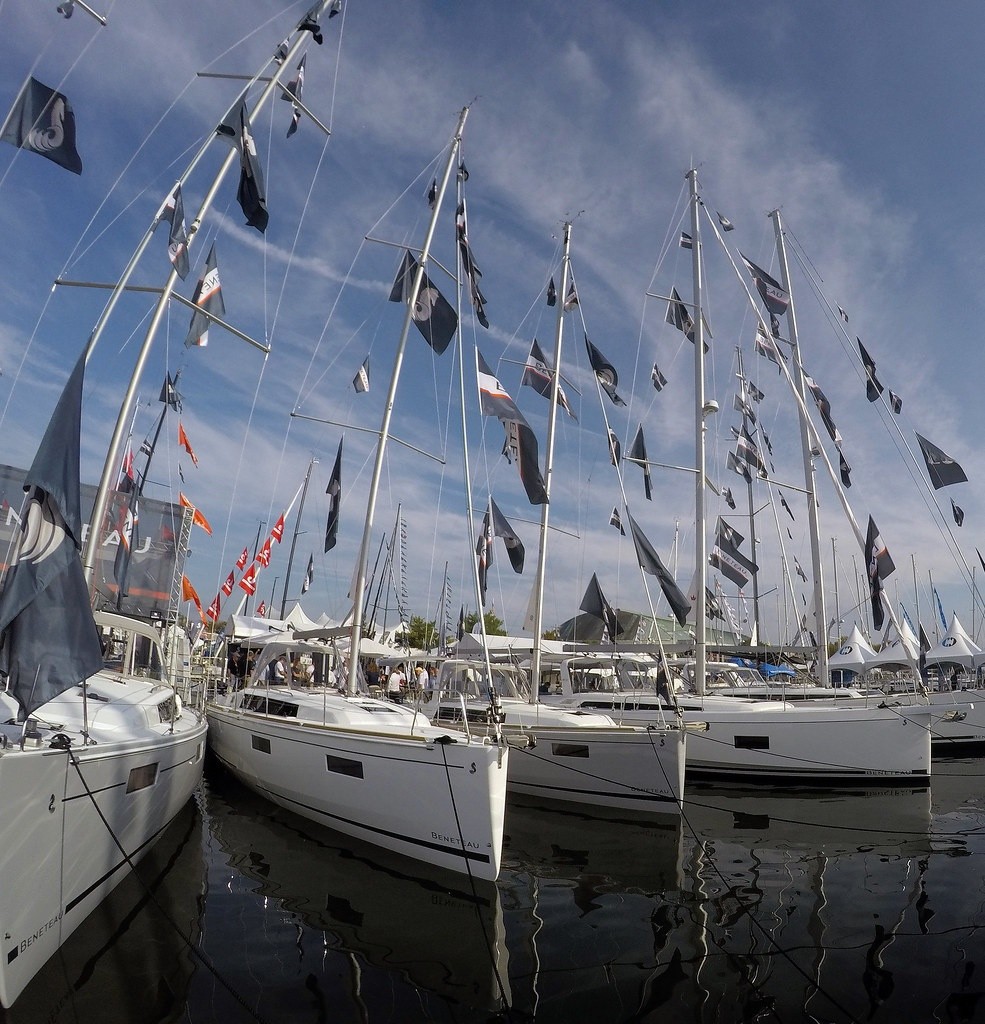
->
[224,601,660,697]
[815,612,982,689]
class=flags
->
[154,0,346,348]
[109,371,211,622]
[917,622,933,690]
[0,76,84,176]
[206,353,371,624]
[389,161,486,356]
[0,329,105,722]
[655,650,672,705]
[665,198,967,631]
[475,337,691,640]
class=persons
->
[430,668,438,688]
[275,657,288,686]
[292,659,306,689]
[415,667,428,690]
[367,663,379,686]
[386,667,401,702]
[339,658,350,690]
[228,651,269,690]
[950,670,959,691]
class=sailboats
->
[209,105,512,905]
[561,168,934,791]
[710,207,985,744]
[419,222,687,830]
[1,0,345,1012]
